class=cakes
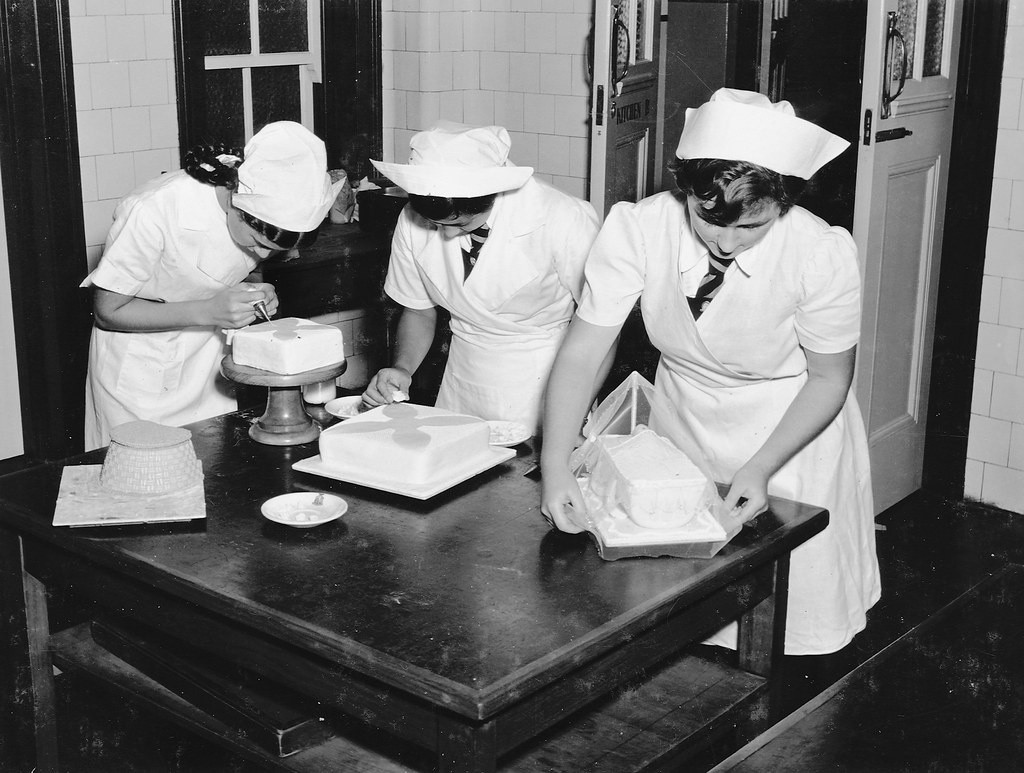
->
[320,404,492,485]
[98,421,199,494]
[232,317,344,374]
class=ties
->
[460,223,490,282]
[686,252,736,321]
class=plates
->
[261,492,349,528]
[325,396,362,418]
[487,421,533,447]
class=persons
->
[78,121,348,453]
[540,88,882,656]
[360,121,621,433]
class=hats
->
[676,87,851,180]
[232,120,347,232]
[369,125,535,198]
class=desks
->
[0,386,828,773]
